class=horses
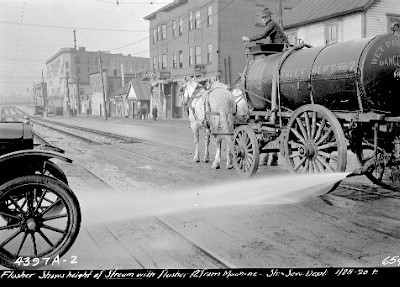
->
[203,76,248,123]
[182,74,238,170]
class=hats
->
[261,8,271,18]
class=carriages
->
[178,33,399,197]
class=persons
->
[240,8,289,44]
[152,105,158,120]
[73,107,78,117]
[142,103,148,120]
